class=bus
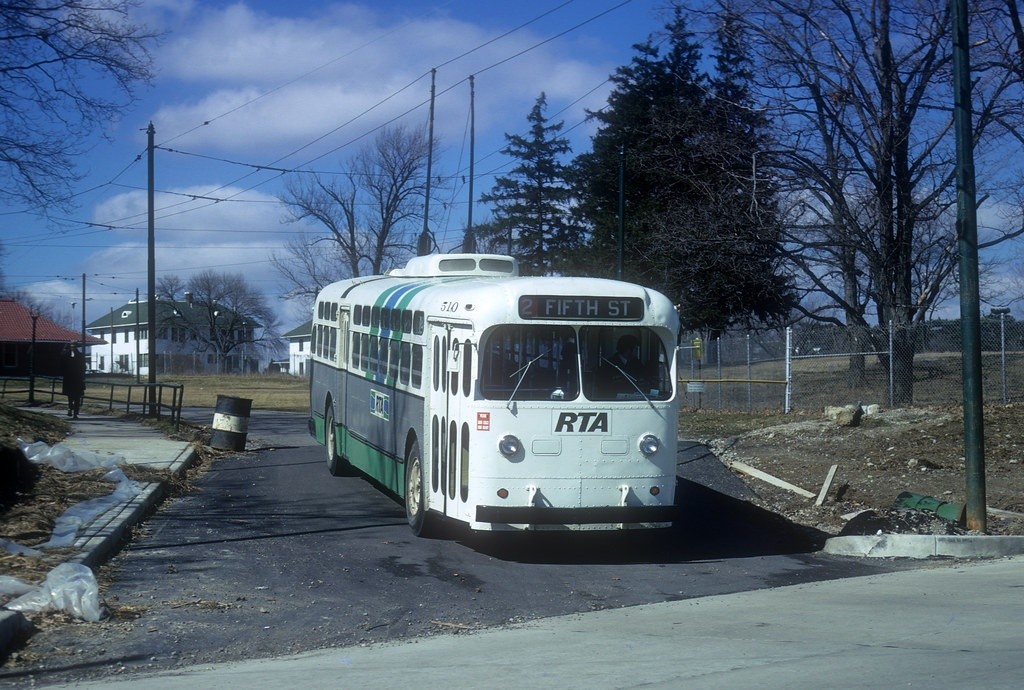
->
[307,252,705,542]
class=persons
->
[62,341,87,420]
[604,334,646,387]
[560,342,577,365]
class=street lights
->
[990,305,1010,404]
[70,301,78,330]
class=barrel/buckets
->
[210,395,253,451]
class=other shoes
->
[73,414,78,419]
[68,409,73,417]
